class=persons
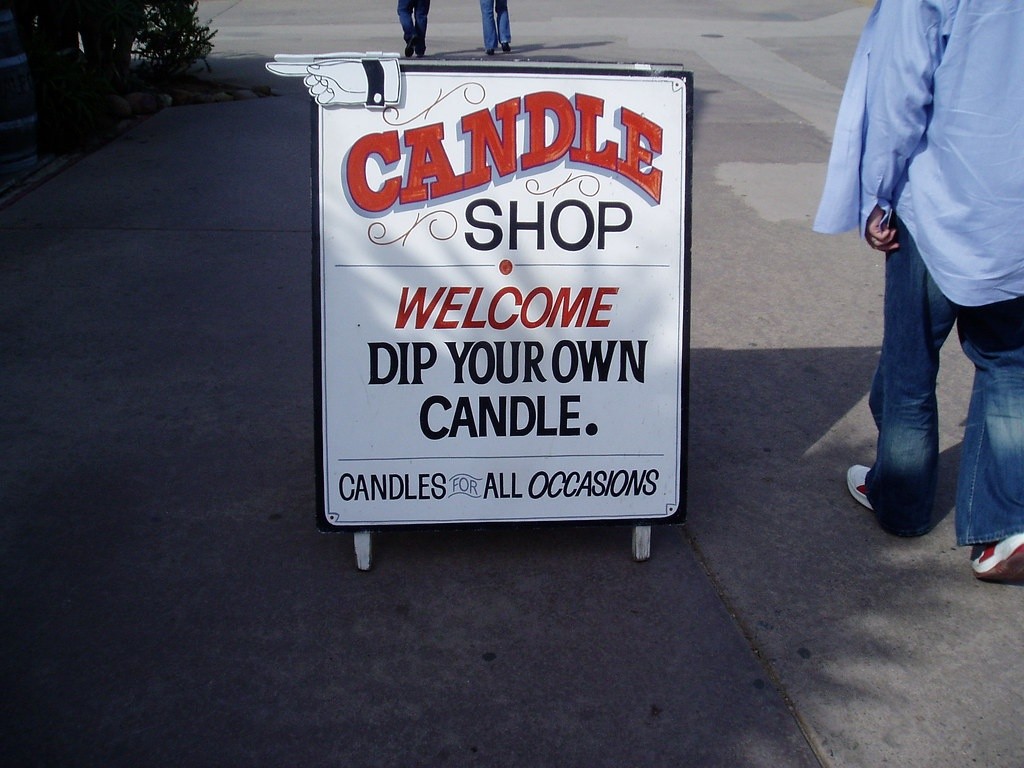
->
[812,0,1024,581]
[480,0,512,54]
[396,0,430,57]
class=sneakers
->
[847,464,874,511]
[971,533,1024,583]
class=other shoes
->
[487,49,494,55]
[405,34,417,58]
[415,38,426,57]
[502,43,511,52]
